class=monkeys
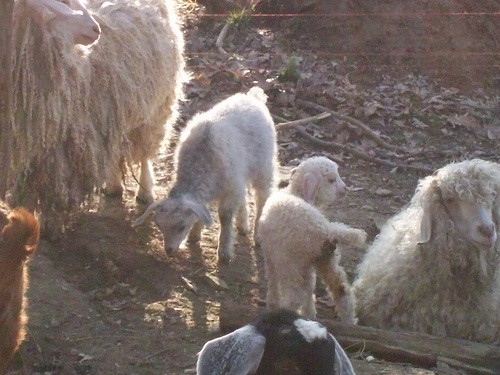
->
[1,0,192,242]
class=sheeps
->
[254,153,369,329]
[1,201,41,375]
[196,307,355,375]
[131,84,282,264]
[350,157,500,347]
